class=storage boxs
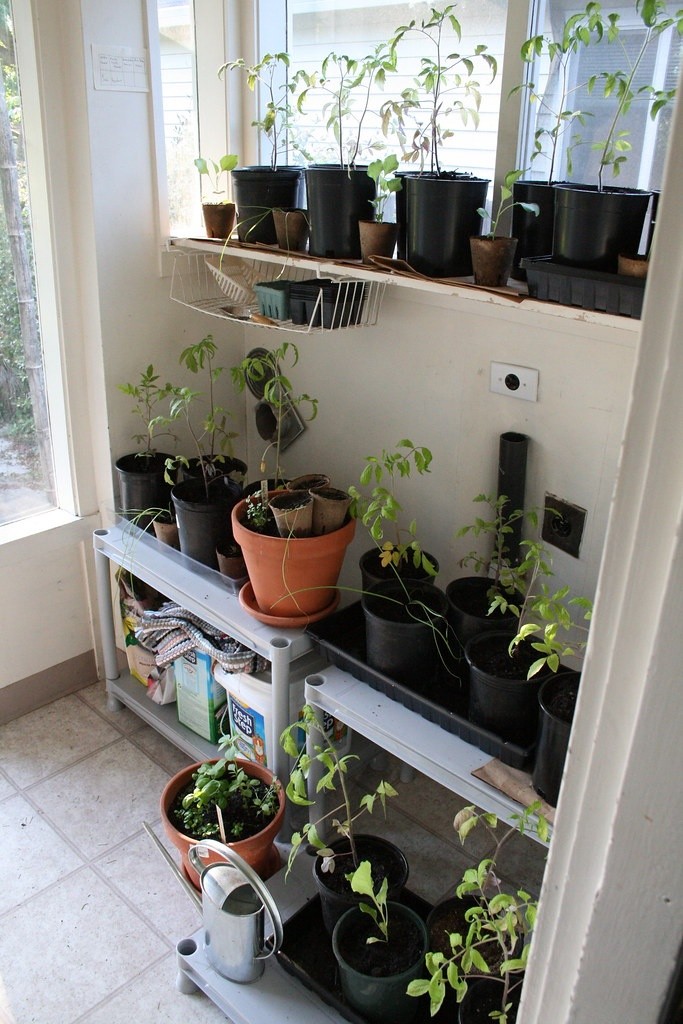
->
[252,280,296,321]
[175,646,228,746]
[288,278,368,328]
[518,253,647,321]
[209,658,351,777]
[119,621,158,686]
[303,599,540,770]
[113,514,247,598]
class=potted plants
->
[279,704,428,1014]
[405,798,552,1024]
[551,0,683,269]
[159,733,287,891]
[269,519,464,679]
[194,154,238,243]
[462,535,561,743]
[468,169,539,288]
[216,51,314,247]
[344,440,440,598]
[443,493,565,690]
[219,203,310,291]
[296,4,498,282]
[357,155,403,263]
[114,363,178,609]
[147,334,246,579]
[230,341,357,628]
[507,1,625,283]
[507,584,594,810]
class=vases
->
[646,188,661,258]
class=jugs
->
[141,819,283,982]
